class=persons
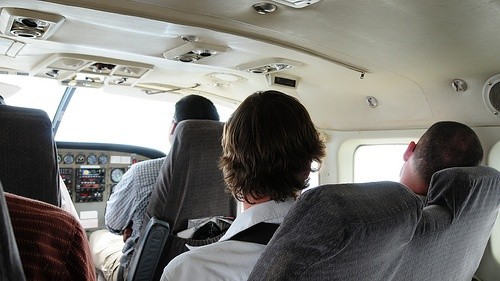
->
[400,121,483,204]
[88,94,219,281]
[0,193,96,281]
[159,90,326,281]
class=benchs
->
[247,165,500,281]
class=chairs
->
[96,117,237,281]
[0,105,61,208]
